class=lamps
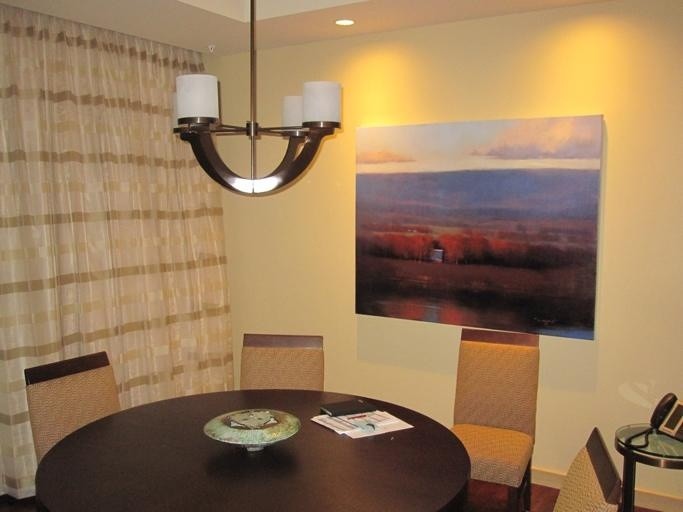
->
[171,1,342,197]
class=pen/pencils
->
[347,415,366,420]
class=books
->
[320,398,377,417]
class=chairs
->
[240,334,325,390]
[24,351,122,464]
[552,427,622,512]
[448,328,539,512]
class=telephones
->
[650,392,683,443]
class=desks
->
[35,390,470,512]
